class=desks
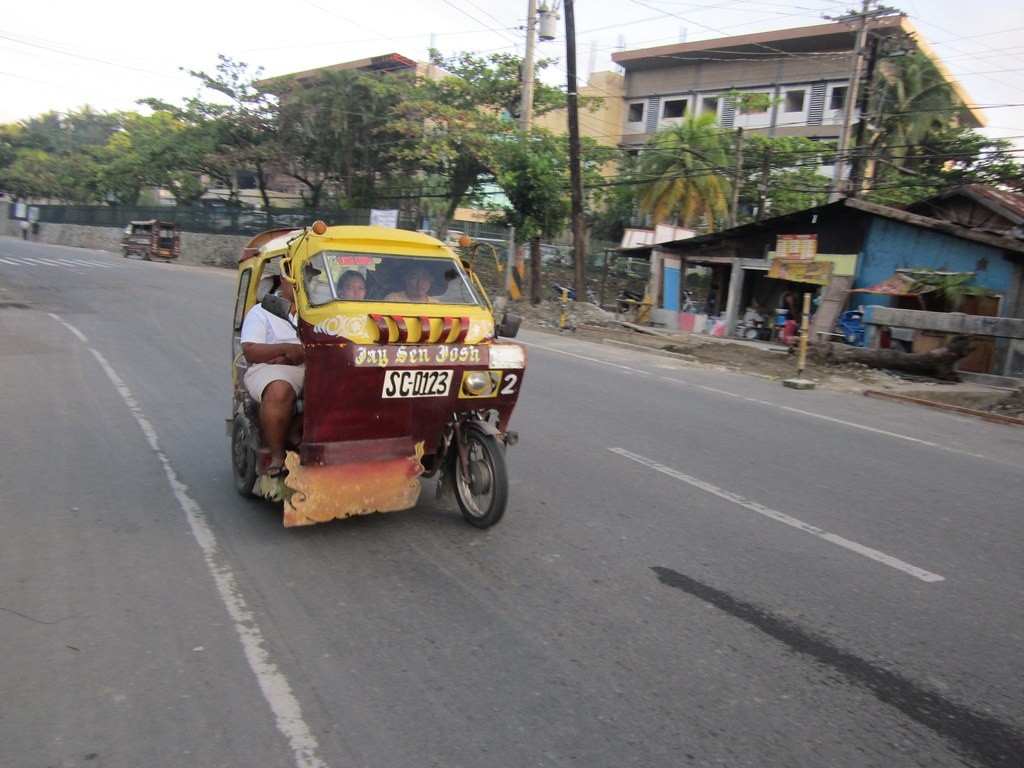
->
[615,299,654,322]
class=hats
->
[268,260,321,294]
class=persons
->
[336,270,367,301]
[240,260,321,477]
[782,294,800,344]
[20,217,41,242]
[382,262,440,303]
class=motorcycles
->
[225,221,527,528]
[121,220,182,262]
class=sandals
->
[268,462,288,478]
